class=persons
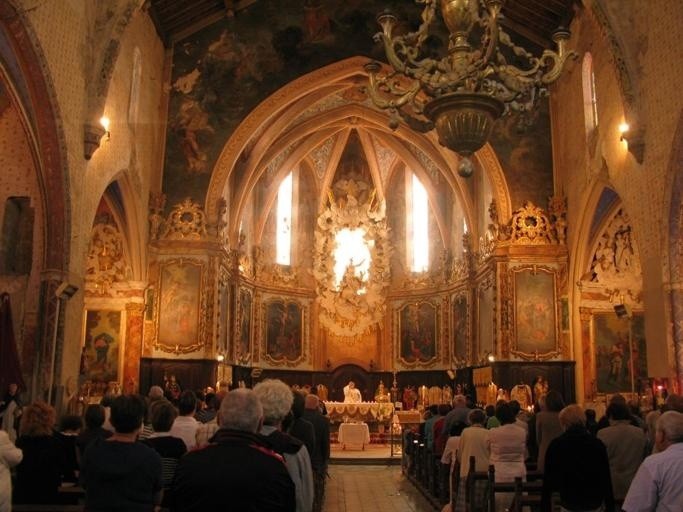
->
[532,374,549,411]
[606,342,624,384]
[409,318,431,359]
[166,373,181,402]
[341,263,366,307]
[374,379,681,511]
[274,304,295,349]
[343,380,362,425]
[1,379,329,512]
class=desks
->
[338,423,370,450]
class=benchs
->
[400,426,622,512]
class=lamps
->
[55,281,79,300]
[357,0,580,177]
[613,304,632,319]
